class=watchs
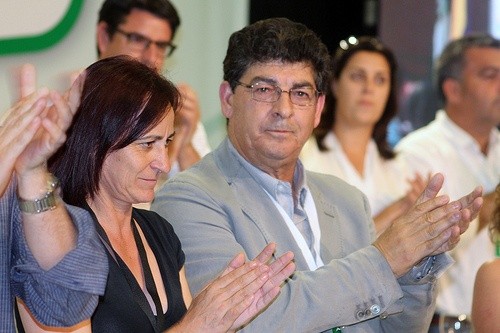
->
[17,172,62,215]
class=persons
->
[47,54,297,333]
[299,35,444,221]
[394,31,500,333]
[0,62,110,333]
[96,0,213,211]
[149,17,484,333]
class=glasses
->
[112,25,177,58]
[229,79,326,107]
